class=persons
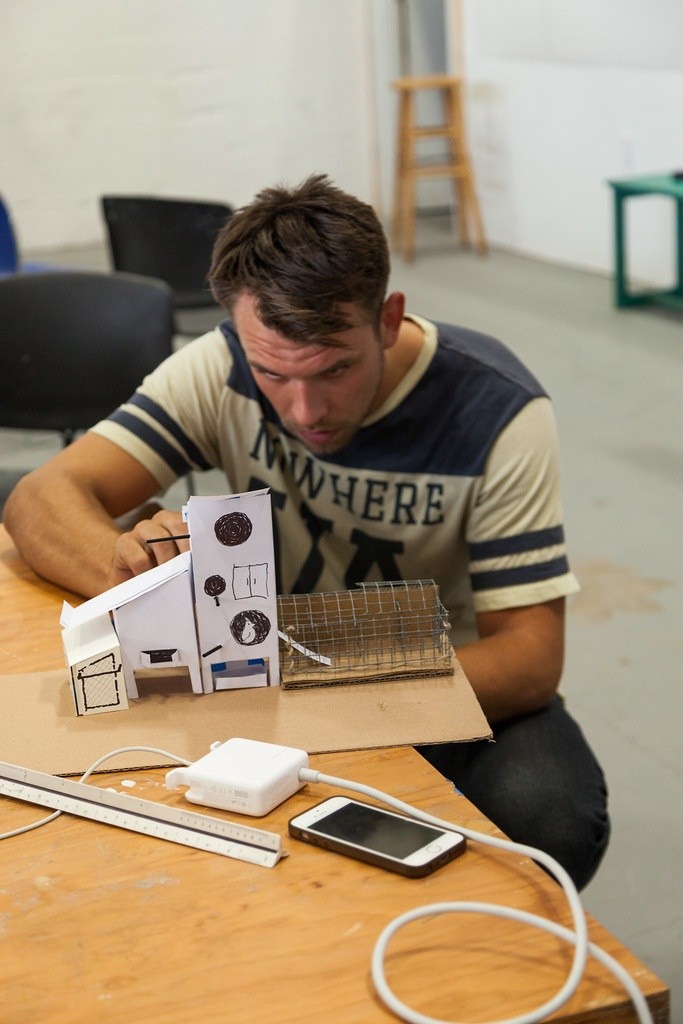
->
[1,173,612,896]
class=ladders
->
[391,77,489,263]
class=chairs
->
[0,266,175,496]
[99,190,241,338]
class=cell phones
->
[288,795,467,880]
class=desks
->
[0,537,669,1024]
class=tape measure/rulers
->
[0,762,290,869]
